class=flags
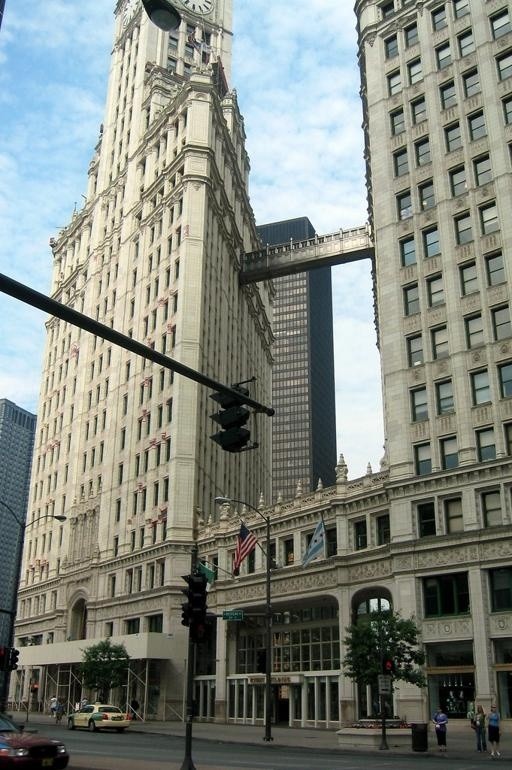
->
[231,523,258,573]
[300,518,328,569]
[198,561,218,597]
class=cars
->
[0,711,69,770]
[69,702,130,732]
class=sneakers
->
[439,748,501,757]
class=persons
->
[81,695,88,707]
[46,695,58,720]
[73,698,81,714]
[430,707,448,752]
[130,695,140,720]
[54,699,68,722]
[473,703,488,753]
[485,703,502,757]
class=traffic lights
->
[30,678,38,693]
[0,647,19,671]
[384,659,393,675]
[209,385,251,450]
[181,572,214,643]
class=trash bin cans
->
[412,723,428,752]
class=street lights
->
[216,497,272,741]
[1,501,66,711]
